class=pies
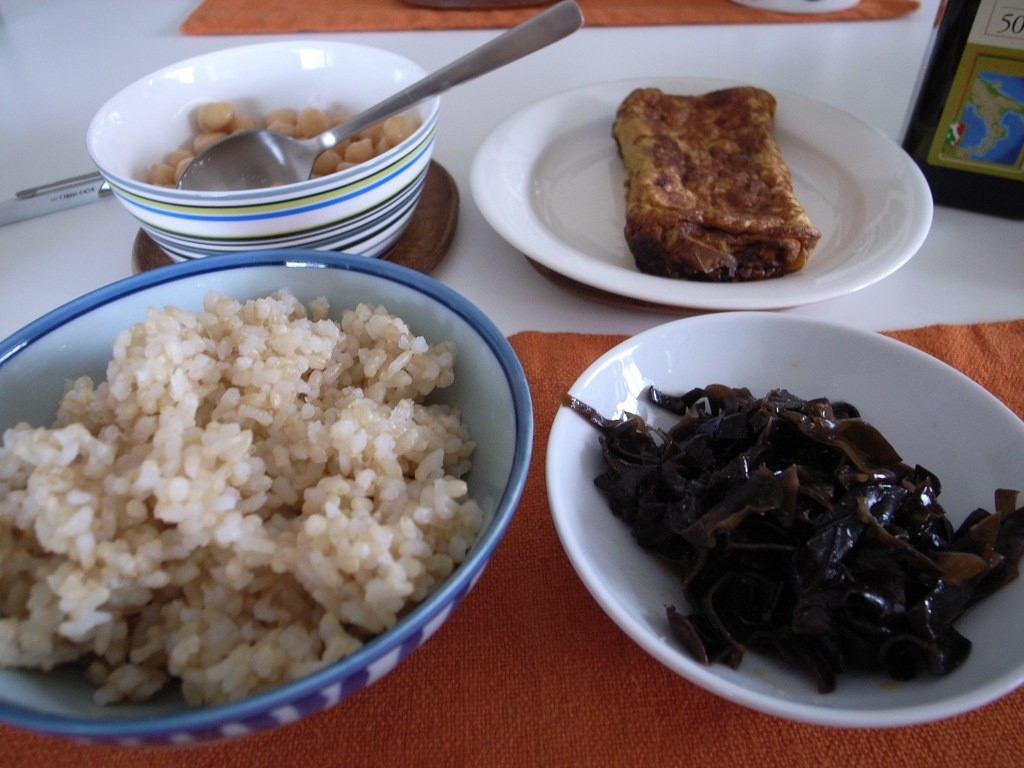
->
[611,88,820,282]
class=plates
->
[467,74,935,309]
[544,312,1024,724]
[528,257,796,317]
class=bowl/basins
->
[0,252,533,745]
[84,41,442,266]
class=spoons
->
[178,0,582,190]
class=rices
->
[0,289,481,707]
